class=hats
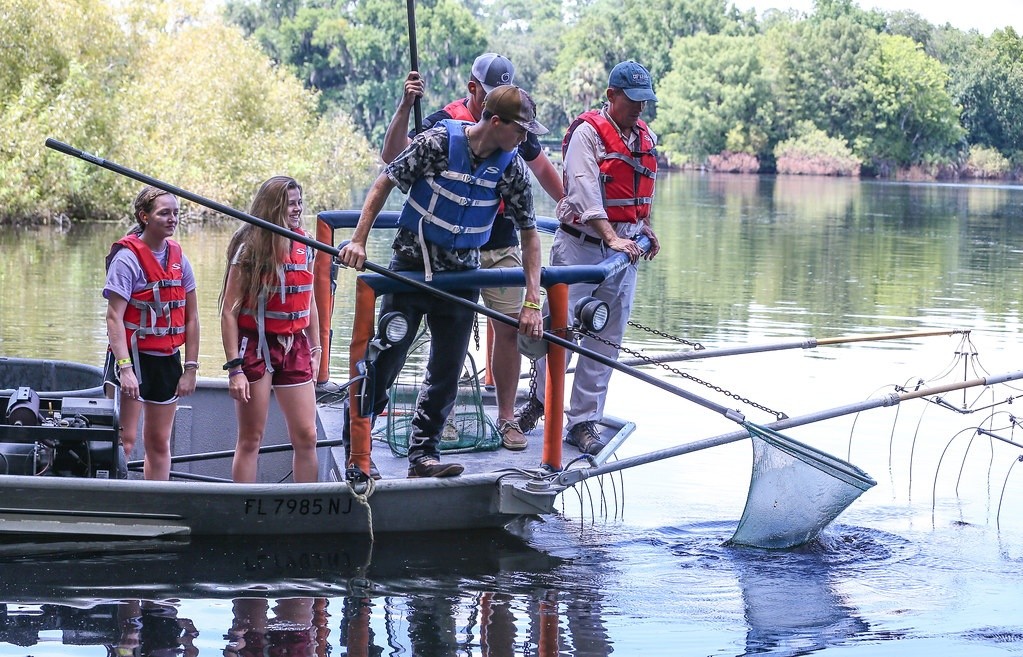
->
[482,85,551,136]
[609,60,660,103]
[471,52,515,92]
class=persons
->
[380,52,564,450]
[220,175,322,485]
[513,60,660,455]
[338,83,542,479]
[102,185,200,480]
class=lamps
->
[364,309,413,369]
[570,294,610,339]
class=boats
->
[0,353,637,544]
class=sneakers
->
[566,419,606,454]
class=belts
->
[558,222,612,248]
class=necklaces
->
[466,126,489,162]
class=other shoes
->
[495,418,528,450]
[439,417,460,444]
[514,396,544,433]
[344,450,383,480]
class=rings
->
[533,328,538,331]
[124,391,129,394]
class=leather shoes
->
[407,455,464,478]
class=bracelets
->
[184,360,199,369]
[523,300,541,310]
[117,363,133,372]
[117,358,132,365]
[223,357,244,370]
[309,345,322,354]
[228,369,243,378]
